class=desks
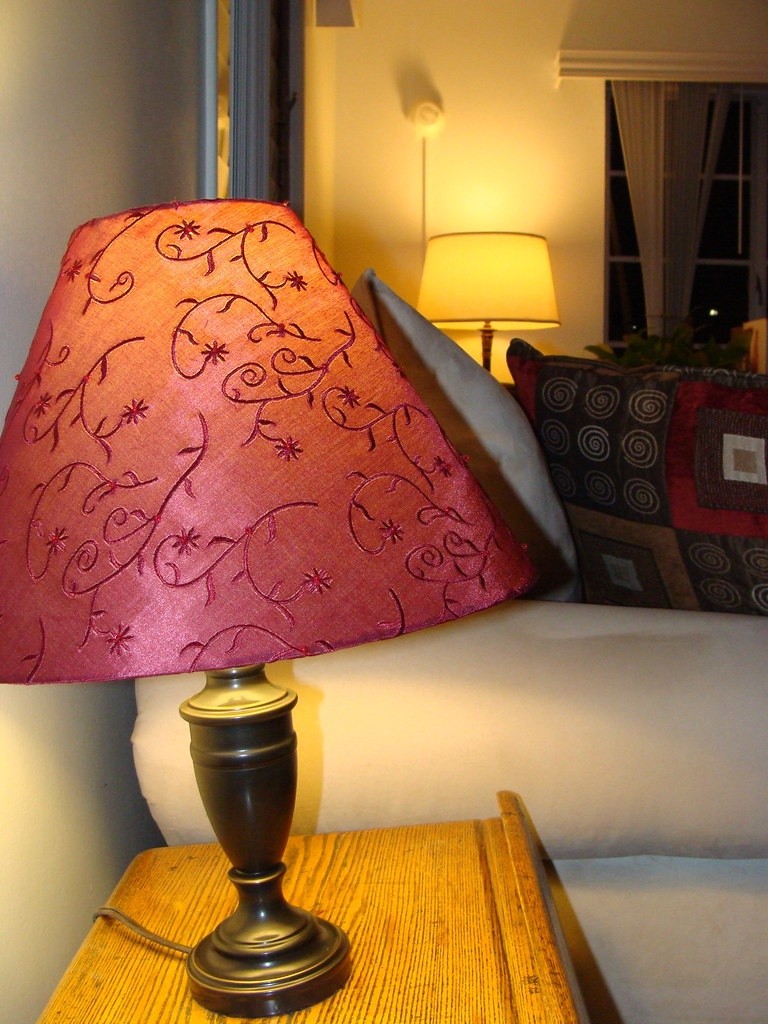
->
[33,787,593,1024]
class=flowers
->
[582,313,754,370]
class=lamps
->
[0,197,535,1017]
[415,232,562,376]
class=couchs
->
[134,600,768,1024]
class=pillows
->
[504,335,768,618]
[349,266,583,606]
